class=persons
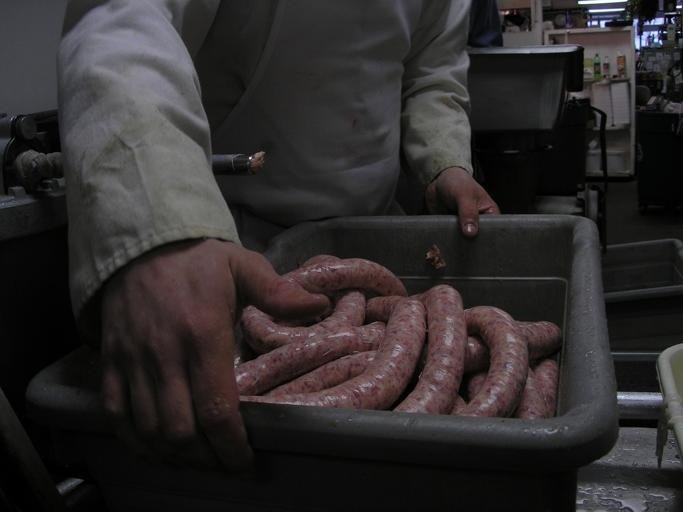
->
[55,0,502,470]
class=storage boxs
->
[25,214,618,508]
[600,238,682,393]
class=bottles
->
[592,52,602,80]
[603,56,610,79]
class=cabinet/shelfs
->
[636,110,683,216]
[543,26,636,179]
